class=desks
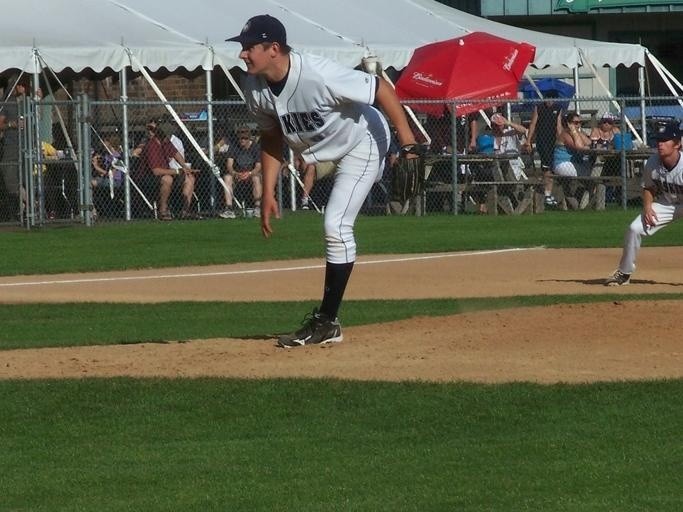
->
[573,148,657,211]
[400,152,520,214]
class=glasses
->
[571,120,583,125]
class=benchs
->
[471,174,620,216]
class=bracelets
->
[176,168,179,174]
[249,171,251,176]
[9,121,11,127]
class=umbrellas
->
[394,30,537,118]
[509,78,576,112]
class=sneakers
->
[278,308,343,349]
[219,210,236,219]
[604,270,631,287]
[181,210,203,220]
[362,55,382,76]
[160,212,173,220]
[301,199,310,211]
[253,208,261,218]
[544,195,560,207]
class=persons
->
[603,120,682,288]
[223,12,423,345]
[361,91,646,215]
[1,70,336,226]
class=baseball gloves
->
[390,145,426,199]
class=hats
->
[156,123,176,135]
[237,127,251,140]
[649,121,681,140]
[491,113,508,126]
[225,14,286,45]
